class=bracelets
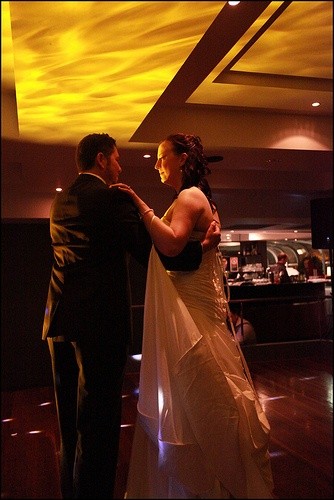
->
[141,208,154,216]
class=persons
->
[300,256,312,275]
[42,133,221,500]
[110,133,274,500]
[274,253,290,280]
[228,310,255,364]
